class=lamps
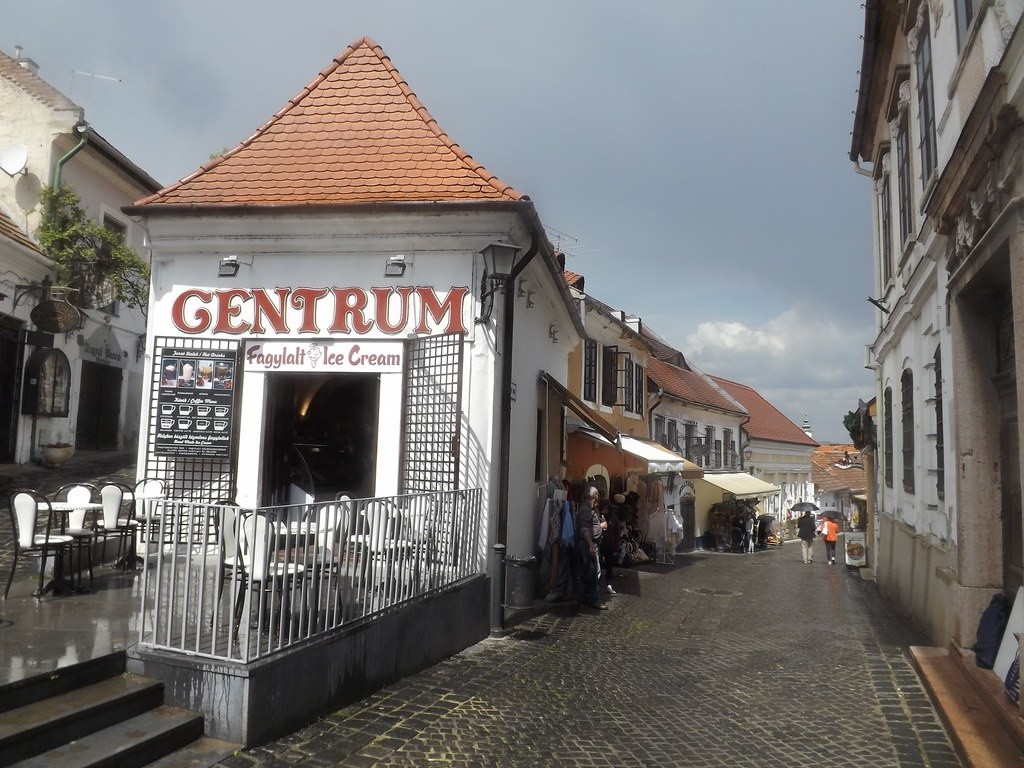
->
[473,235,523,325]
[384,255,414,278]
[217,254,252,278]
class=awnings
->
[544,371,624,455]
[566,431,704,480]
[701,472,781,499]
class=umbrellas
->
[822,511,848,521]
[790,502,820,511]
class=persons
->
[821,517,840,564]
[744,514,755,554]
[547,474,566,498]
[797,511,817,564]
[561,479,638,610]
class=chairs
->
[133,478,171,560]
[3,488,76,603]
[44,483,103,592]
[90,481,139,574]
[213,490,440,632]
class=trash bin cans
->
[639,541,657,566]
[503,553,539,612]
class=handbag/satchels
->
[622,528,656,564]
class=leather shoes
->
[590,600,608,610]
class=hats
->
[614,494,625,503]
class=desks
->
[262,522,334,638]
[98,492,168,570]
[360,509,427,597]
[36,501,104,599]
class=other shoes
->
[808,558,812,563]
[828,561,832,566]
[607,586,616,594]
[803,560,807,564]
[831,557,835,563]
